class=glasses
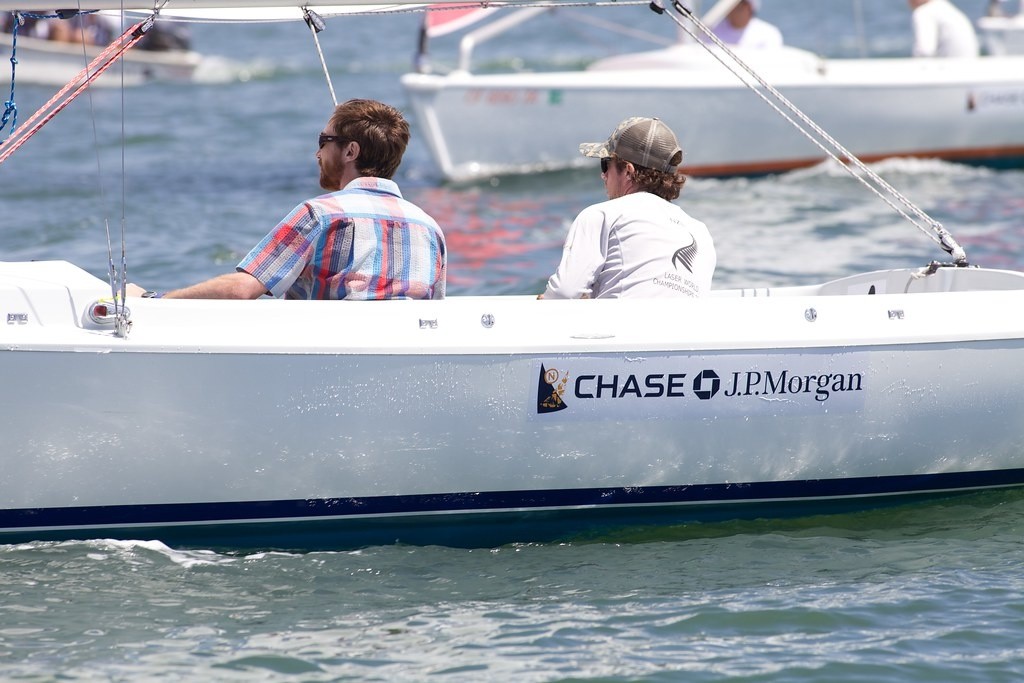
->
[319,132,344,149]
[601,156,620,173]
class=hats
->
[579,116,681,174]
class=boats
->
[0,0,1023,551]
[398,0,1024,186]
[0,29,196,89]
[976,8,1024,59]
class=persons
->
[905,0,982,58]
[535,115,717,298]
[0,7,194,51]
[158,99,448,300]
[700,0,785,45]
